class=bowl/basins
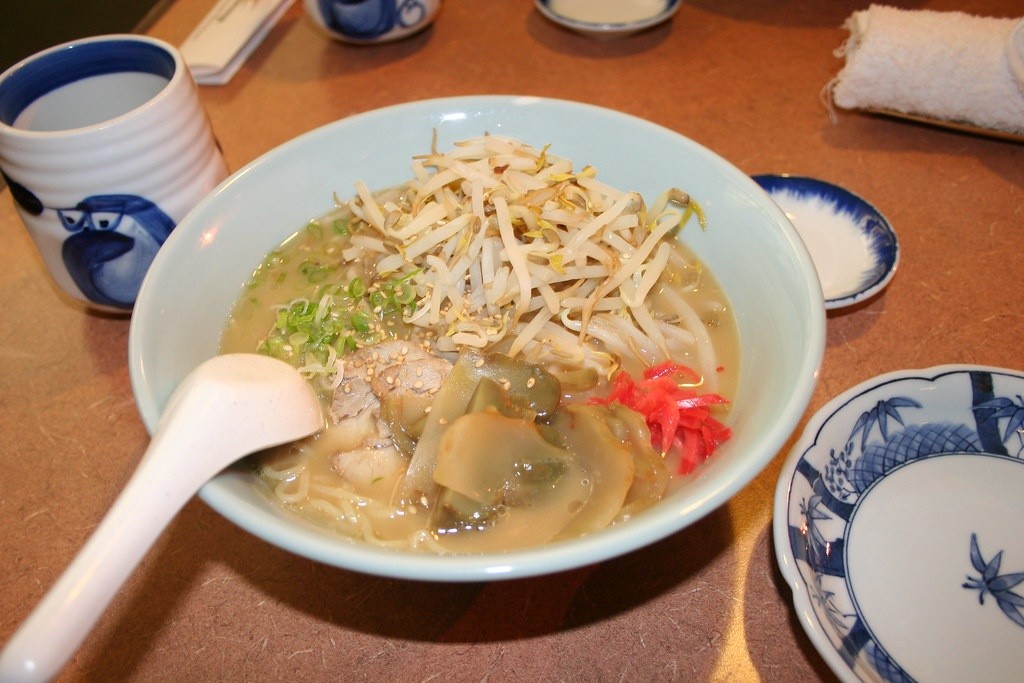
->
[128,94,827,581]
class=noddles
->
[219,192,741,553]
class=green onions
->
[243,213,417,376]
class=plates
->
[752,175,900,310]
[774,364,1024,683]
[534,0,682,39]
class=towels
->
[820,3,1024,134]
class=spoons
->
[0,352,325,683]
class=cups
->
[0,35,231,317]
[303,0,439,45]
